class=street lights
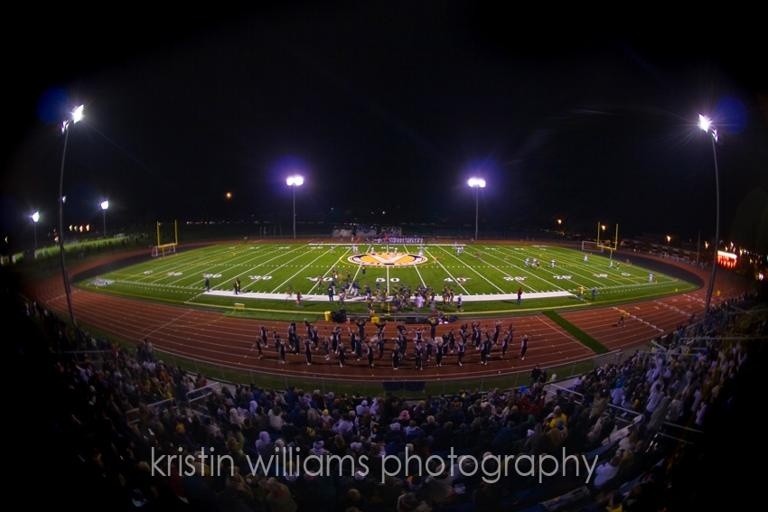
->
[467,176,487,240]
[51,101,87,333]
[697,114,722,318]
[286,173,307,239]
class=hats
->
[207,388,414,480]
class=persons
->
[0,235,768,512]
